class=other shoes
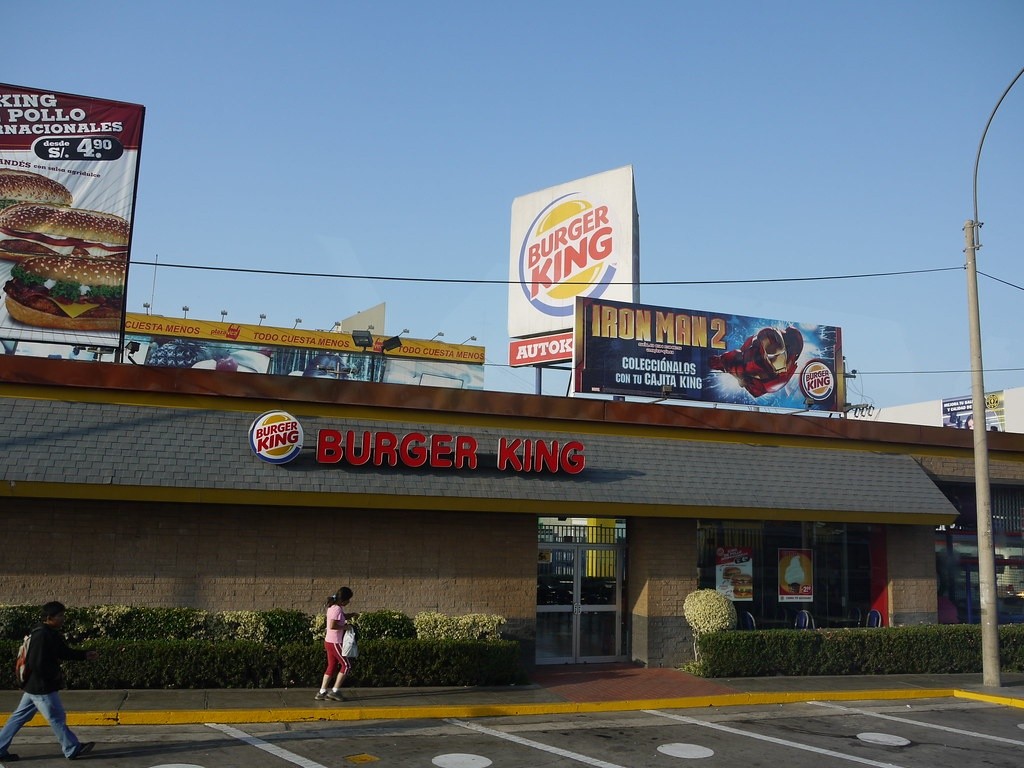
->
[314,690,332,701]
[326,689,343,701]
[68,742,95,760]
[0,751,19,762]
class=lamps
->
[125,342,141,364]
[381,335,402,353]
[293,318,302,328]
[221,309,227,322]
[787,397,815,414]
[259,314,266,326]
[352,330,374,352]
[183,306,189,319]
[328,321,341,332]
[461,335,476,345]
[143,303,150,315]
[367,325,374,331]
[649,386,673,403]
[399,329,409,337]
[429,332,444,341]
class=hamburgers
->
[0,167,131,331]
[722,567,753,598]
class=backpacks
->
[16,627,60,683]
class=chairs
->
[866,609,883,628]
[739,611,756,631]
[794,610,815,630]
[773,608,787,628]
[845,607,862,628]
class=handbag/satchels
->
[342,626,358,657]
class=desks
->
[757,619,792,629]
[815,616,859,628]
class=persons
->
[315,587,360,701]
[0,601,96,762]
[965,414,973,430]
